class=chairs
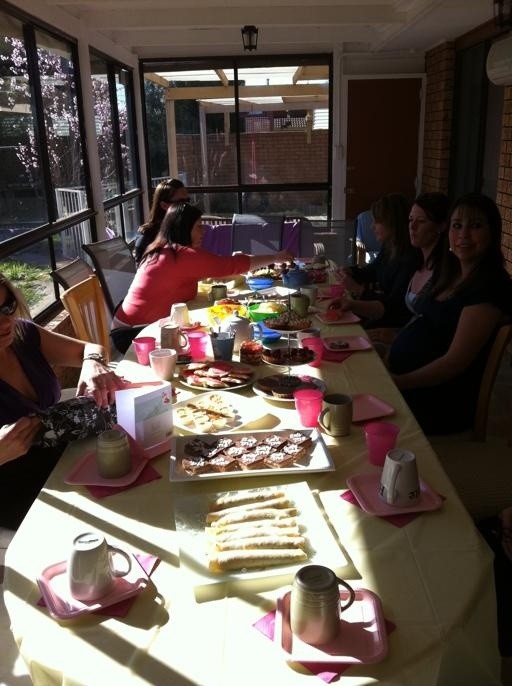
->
[427,324,509,517]
[50,237,139,368]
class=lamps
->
[240,23,261,50]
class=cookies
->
[181,360,252,389]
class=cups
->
[362,421,400,467]
[210,332,235,361]
[301,338,323,366]
[299,287,313,304]
[293,389,323,426]
[376,448,425,508]
[290,294,309,316]
[318,393,352,436]
[130,302,192,382]
[187,331,206,360]
[68,532,133,602]
[211,285,227,303]
[290,565,356,646]
[97,429,132,479]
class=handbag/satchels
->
[30,396,118,447]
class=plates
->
[170,253,397,435]
[110,420,176,460]
[346,470,442,516]
[65,452,150,488]
[274,584,390,665]
[36,544,154,622]
[172,481,349,588]
[168,426,336,483]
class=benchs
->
[182,217,318,256]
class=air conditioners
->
[485,36,511,88]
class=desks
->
[1,258,491,681]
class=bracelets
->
[82,353,109,367]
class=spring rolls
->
[206,490,308,574]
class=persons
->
[133,179,186,268]
[0,269,124,533]
[384,195,511,436]
[338,192,410,328]
[329,192,462,356]
[110,199,293,355]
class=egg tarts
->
[176,394,232,431]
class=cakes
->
[182,430,312,475]
